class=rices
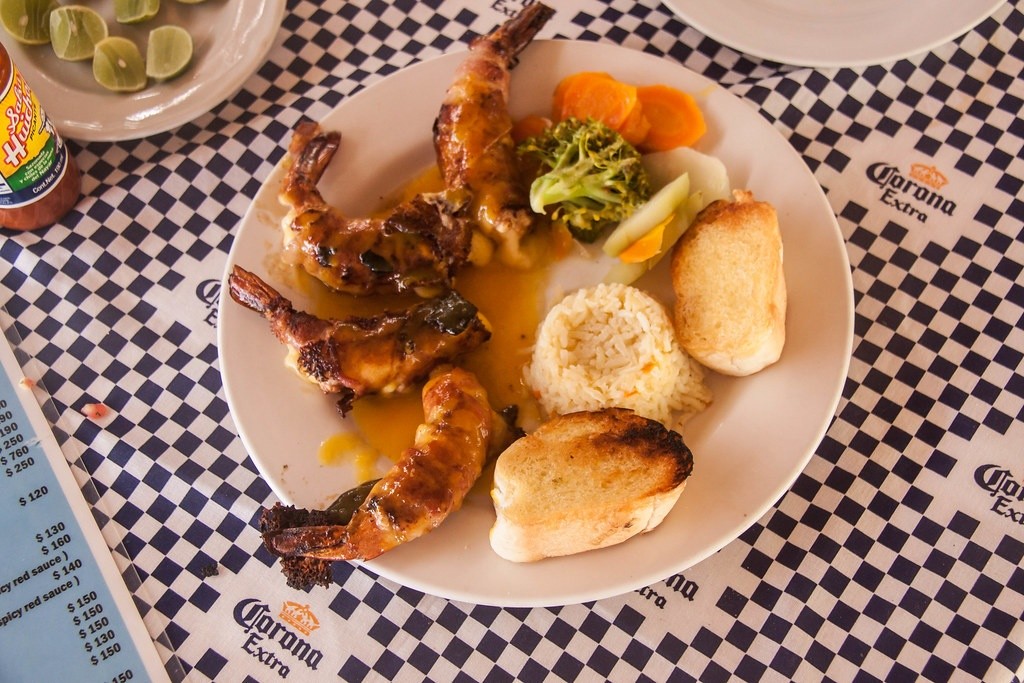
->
[521,283,710,432]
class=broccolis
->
[516,118,650,240]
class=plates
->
[0,0,288,143]
[661,0,1010,68]
[217,40,854,609]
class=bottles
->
[0,41,81,231]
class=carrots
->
[551,74,706,152]
